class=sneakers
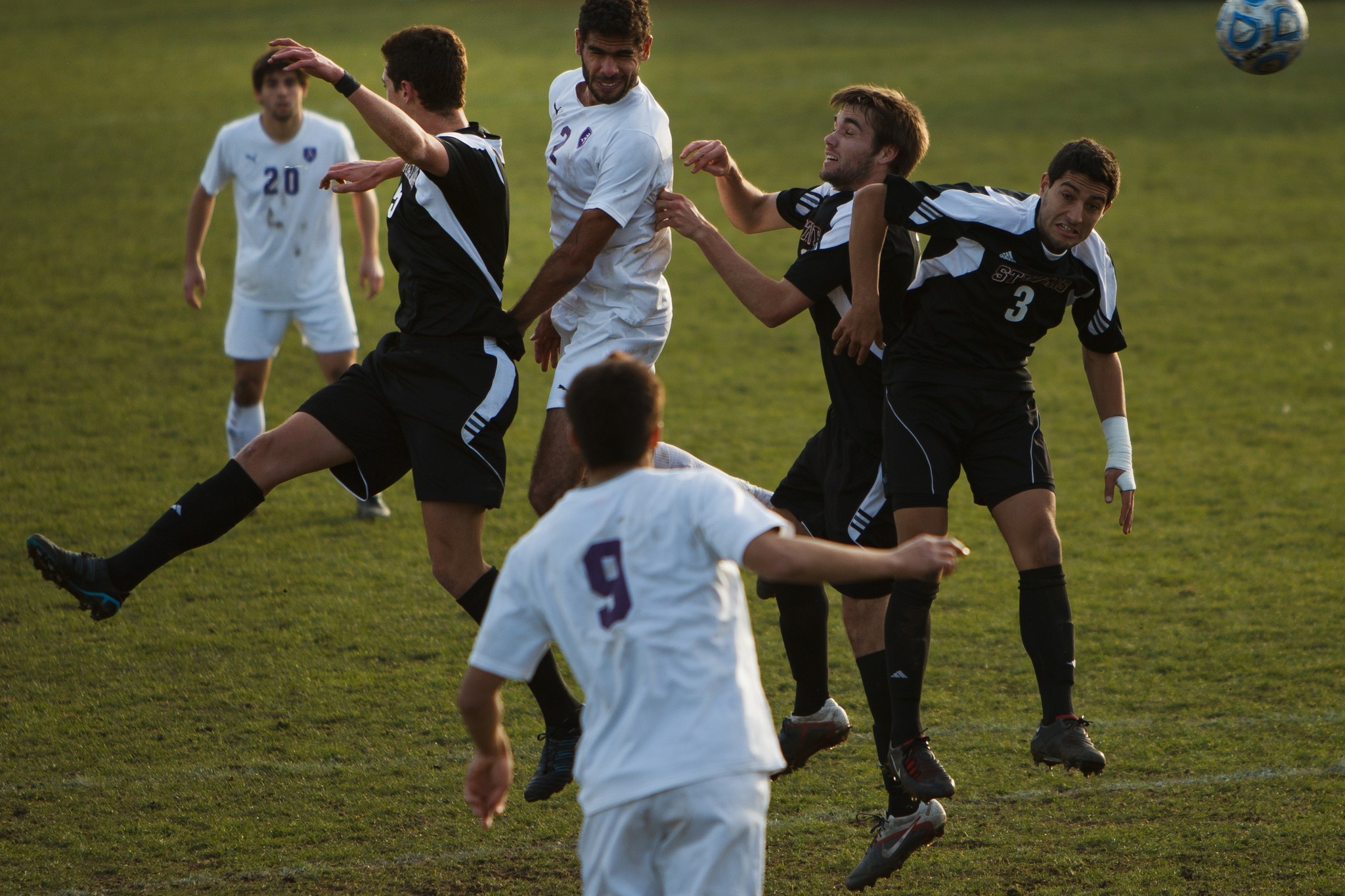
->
[1029,715,1107,778]
[522,701,587,804]
[886,732,958,807]
[356,493,391,521]
[24,532,130,622]
[769,698,853,781]
[843,798,949,892]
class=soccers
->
[1216,0,1308,77]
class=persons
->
[172,48,397,522]
[648,83,952,892]
[442,342,974,896]
[487,0,816,567]
[836,132,1140,796]
[27,27,587,804]
